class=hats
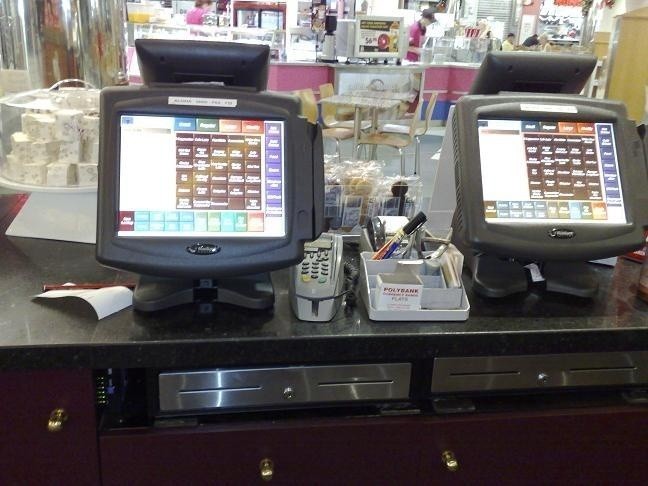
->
[421,9,435,20]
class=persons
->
[405,8,438,63]
[501,32,541,51]
[185,0,213,25]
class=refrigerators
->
[232,0,286,31]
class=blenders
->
[320,13,338,63]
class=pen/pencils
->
[373,213,428,260]
[41,282,136,291]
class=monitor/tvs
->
[452,91,646,297]
[134,38,270,92]
[466,53,599,97]
[236,9,258,28]
[95,82,327,311]
[259,9,284,30]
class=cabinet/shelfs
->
[95,408,648,486]
[1,369,99,486]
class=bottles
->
[545,43,551,54]
[389,20,399,51]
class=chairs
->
[291,82,440,177]
